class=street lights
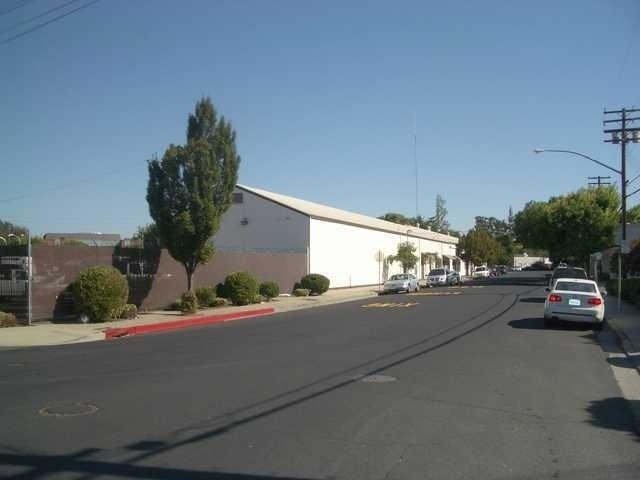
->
[534,148,627,277]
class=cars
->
[472,264,522,279]
[545,263,606,330]
[383,273,420,293]
[425,268,459,286]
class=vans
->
[115,261,156,297]
[0,255,27,297]
